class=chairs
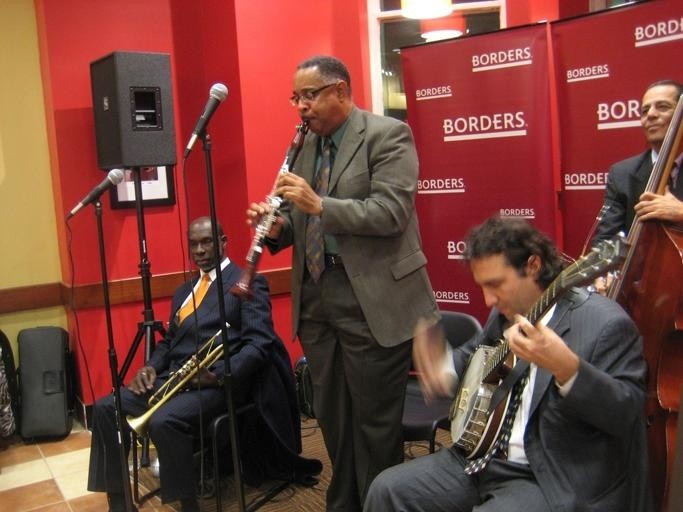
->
[401,310,483,458]
[131,335,299,512]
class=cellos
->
[582,91,682,512]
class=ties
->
[179,275,210,324]
[304,137,332,286]
[464,366,529,477]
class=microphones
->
[68,169,126,218]
[185,83,228,157]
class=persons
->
[586,79,683,297]
[86,217,276,512]
[364,217,647,512]
[246,55,446,512]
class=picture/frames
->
[108,164,175,209]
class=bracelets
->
[319,197,323,217]
[216,373,224,389]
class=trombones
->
[124,322,231,435]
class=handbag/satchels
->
[0,326,71,441]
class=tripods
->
[118,168,167,467]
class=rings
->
[141,372,147,376]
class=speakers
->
[85,51,177,171]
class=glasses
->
[289,81,337,106]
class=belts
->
[319,253,343,269]
